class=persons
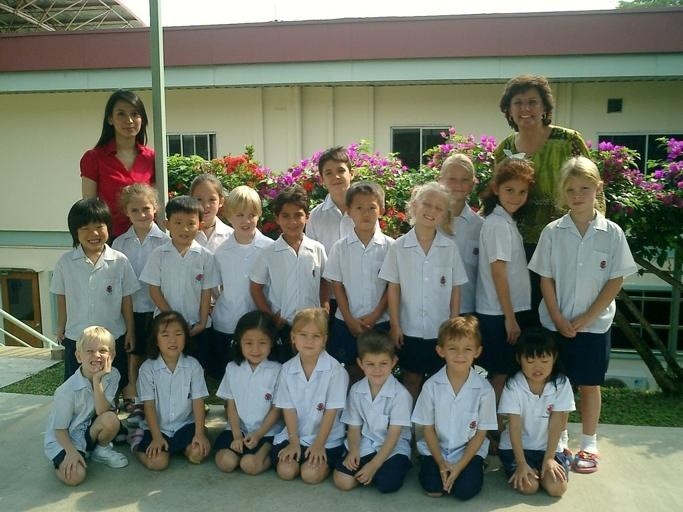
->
[273,307,350,484]
[207,184,275,421]
[50,197,143,404]
[249,185,329,364]
[474,149,536,454]
[482,73,606,312]
[80,89,157,413]
[138,194,222,381]
[379,181,469,399]
[332,327,413,494]
[498,327,577,498]
[410,315,499,502]
[304,144,384,322]
[442,154,485,319]
[125,310,209,470]
[189,173,234,316]
[330,181,396,397]
[44,326,128,486]
[214,311,283,476]
[110,181,172,427]
[526,155,639,472]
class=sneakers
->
[90,445,128,467]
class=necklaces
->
[201,223,215,229]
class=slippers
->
[574,449,602,472]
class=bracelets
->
[288,431,298,438]
[197,318,207,326]
